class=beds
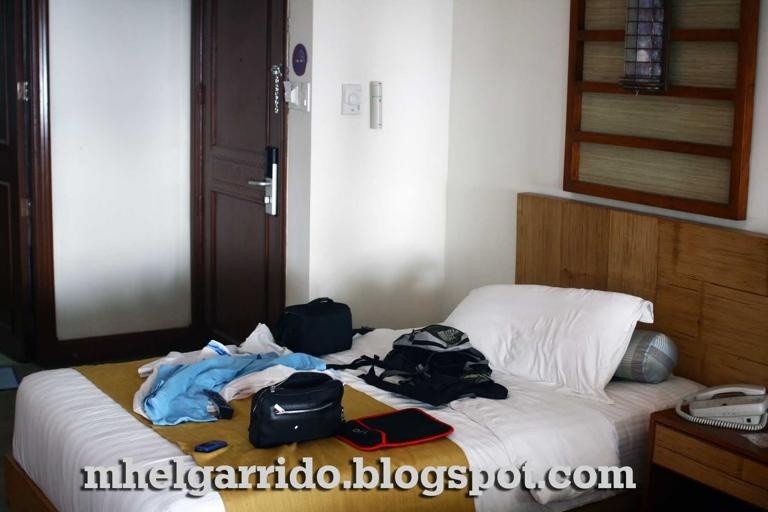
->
[6,191,767,511]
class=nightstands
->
[638,402,767,512]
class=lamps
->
[616,0,670,101]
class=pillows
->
[442,282,681,410]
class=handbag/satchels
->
[274,296,356,360]
[247,371,349,447]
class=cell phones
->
[195,440,227,452]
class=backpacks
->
[366,324,510,408]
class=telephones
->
[689,384,768,425]
